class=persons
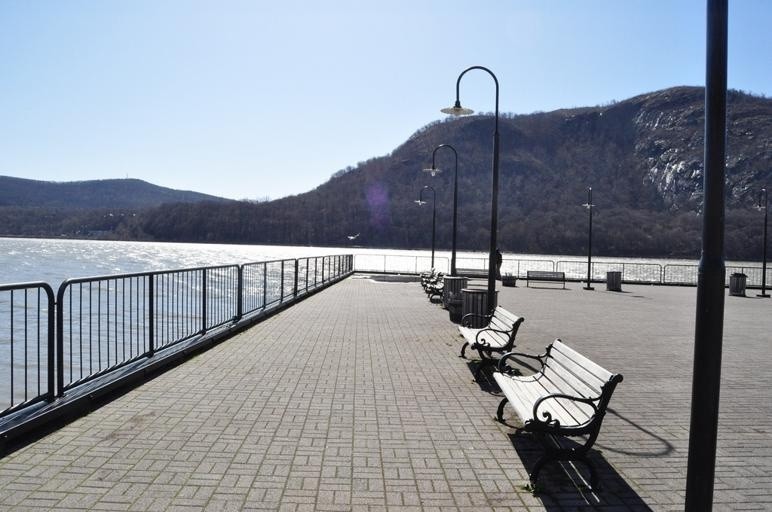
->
[496,248,502,280]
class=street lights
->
[415,66,499,312]
[757,188,770,297]
[583,187,595,290]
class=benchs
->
[458,306,524,368]
[420,265,450,302]
[524,269,567,288]
[493,340,624,492]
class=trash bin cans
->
[607,272,621,290]
[729,273,746,296]
[443,275,468,308]
[461,288,500,329]
